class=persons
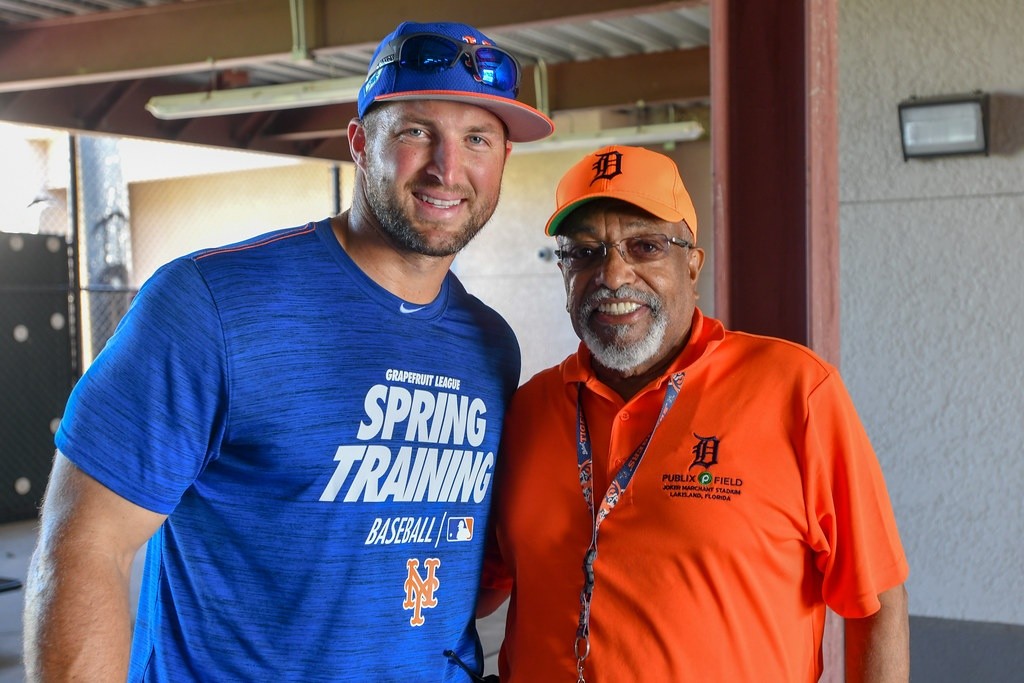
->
[477,144,909,683]
[23,21,555,683]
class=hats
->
[545,144,698,245]
[357,20,555,144]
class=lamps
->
[897,89,991,162]
[508,100,706,160]
[146,75,367,121]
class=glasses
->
[554,233,695,270]
[365,32,523,99]
